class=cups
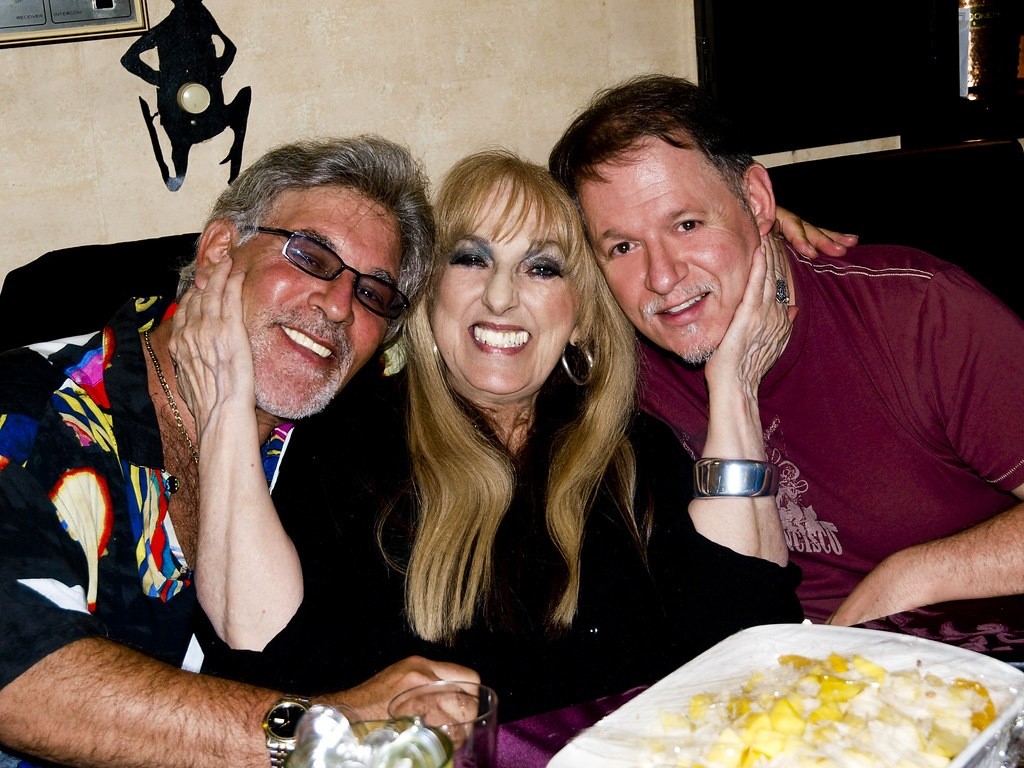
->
[288,720,455,768]
[387,680,498,768]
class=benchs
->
[0,142,1024,331]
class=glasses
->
[239,225,410,323]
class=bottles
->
[958,0,991,140]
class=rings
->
[775,279,791,303]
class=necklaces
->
[143,333,202,479]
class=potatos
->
[654,651,996,768]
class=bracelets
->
[692,458,780,498]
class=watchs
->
[262,689,312,768]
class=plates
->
[546,623,1024,767]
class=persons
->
[0,134,857,768]
[168,149,805,724]
[549,75,1024,637]
